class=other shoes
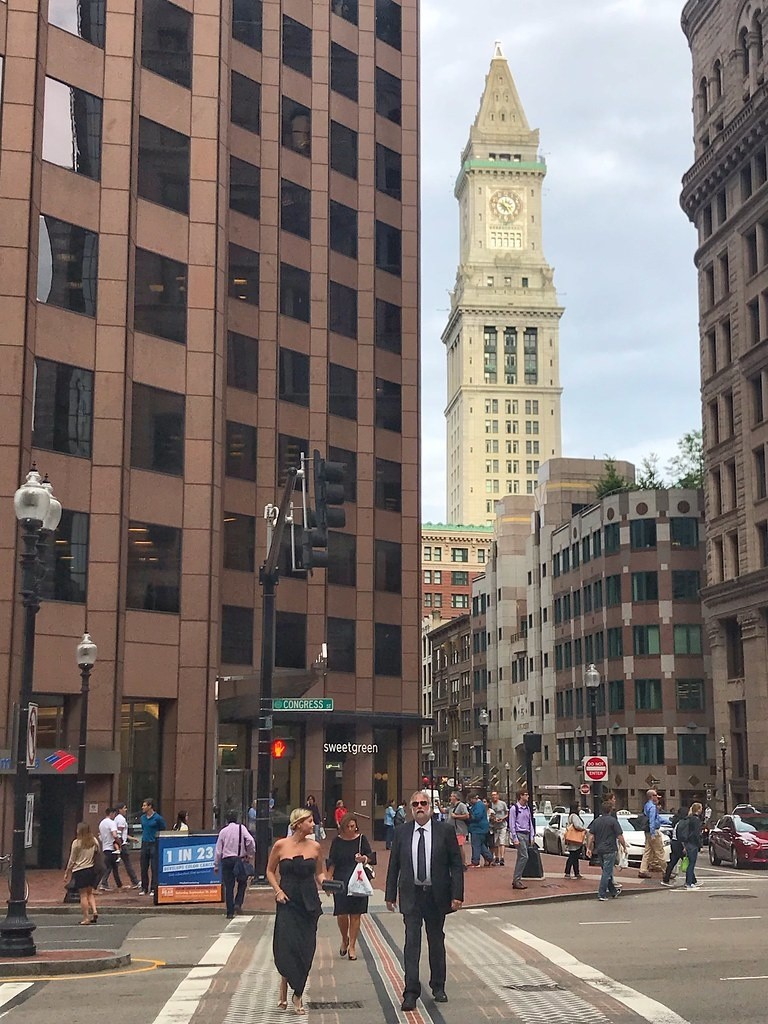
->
[116,857,123,862]
[226,906,244,919]
[565,874,586,881]
[112,850,121,855]
[101,881,154,896]
[340,937,357,960]
[638,872,704,887]
[76,913,98,925]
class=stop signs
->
[584,754,608,781]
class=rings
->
[459,905,461,908]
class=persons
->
[435,791,509,871]
[384,790,464,1011]
[334,800,348,829]
[600,793,618,819]
[173,810,190,831]
[214,808,256,918]
[324,812,377,961]
[139,797,166,896]
[565,801,587,880]
[384,798,407,850]
[509,789,535,890]
[638,790,711,889]
[98,802,142,891]
[64,822,101,925]
[304,795,323,844]
[586,801,627,901]
[267,808,337,1014]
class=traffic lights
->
[272,736,297,760]
[300,508,333,574]
[312,448,348,530]
[422,775,431,785]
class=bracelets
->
[275,890,283,896]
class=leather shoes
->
[402,989,449,1010]
[513,882,528,889]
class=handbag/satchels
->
[233,856,255,877]
[394,812,406,826]
[456,833,466,845]
[319,827,327,839]
[566,824,585,845]
[95,851,106,874]
[637,816,649,831]
[323,863,375,896]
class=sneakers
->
[599,888,622,901]
[467,857,506,868]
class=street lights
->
[479,704,492,801]
[0,460,62,957]
[585,663,601,822]
[450,735,461,796]
[78,625,98,836]
[504,761,512,808]
[718,734,729,815]
[427,747,435,812]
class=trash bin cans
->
[126,825,144,850]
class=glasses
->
[411,801,428,807]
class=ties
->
[418,828,427,882]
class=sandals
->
[278,990,305,1015]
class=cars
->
[506,807,676,868]
[708,803,768,869]
